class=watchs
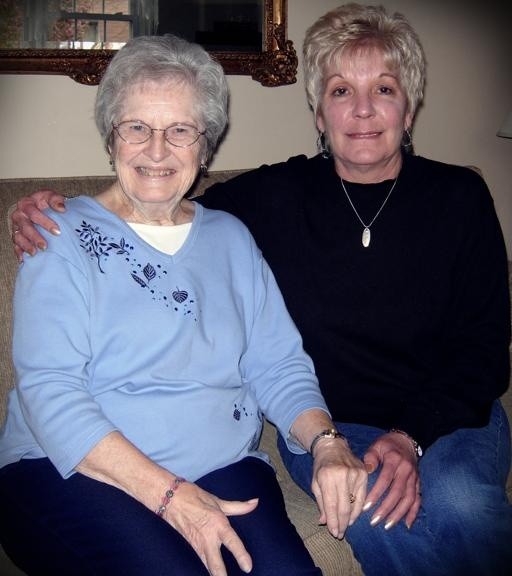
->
[386,427,426,460]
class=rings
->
[348,493,357,505]
[412,491,423,499]
[11,229,21,243]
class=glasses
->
[111,120,206,147]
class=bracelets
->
[153,474,188,521]
[309,426,351,460]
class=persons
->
[6,2,511,576]
[2,32,370,576]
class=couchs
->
[0,167,361,575]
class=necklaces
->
[332,155,404,250]
